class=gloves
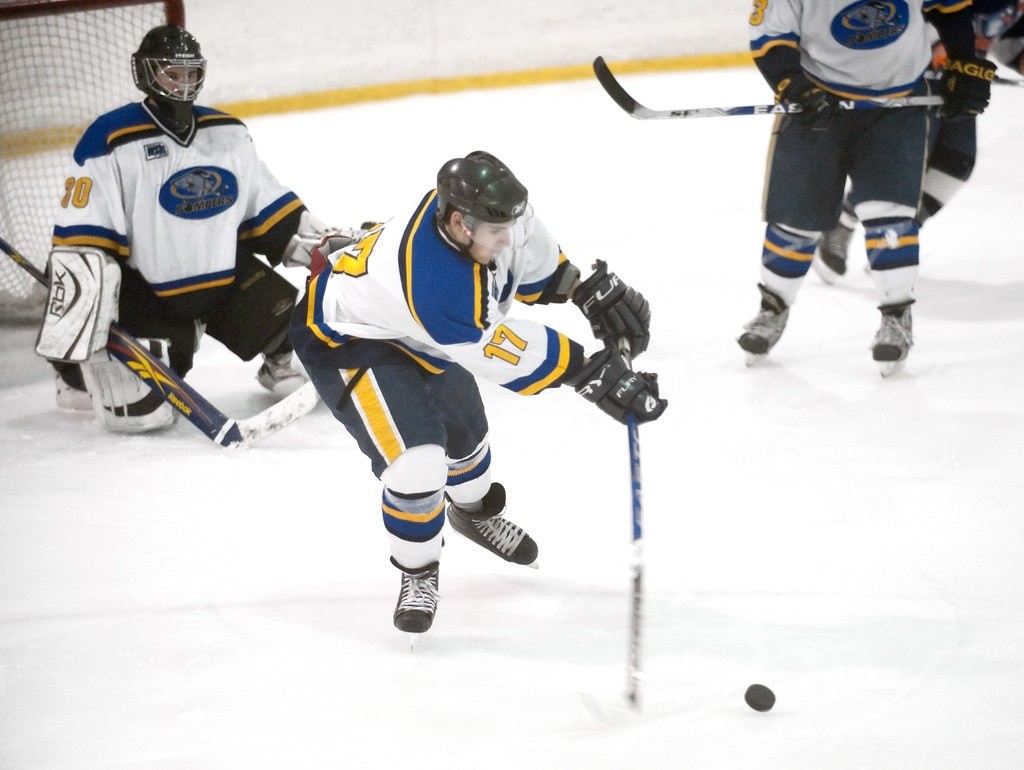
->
[573,259,650,359]
[775,75,837,131]
[562,347,667,424]
[927,51,999,122]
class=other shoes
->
[255,353,306,398]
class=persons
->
[289,149,670,654]
[736,0,1024,378]
[34,22,384,434]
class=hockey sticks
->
[590,53,946,125]
[993,73,1024,89]
[1,234,324,451]
[592,253,650,713]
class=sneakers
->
[389,536,444,652]
[813,204,862,284]
[738,282,788,366]
[444,482,540,569]
[872,299,915,379]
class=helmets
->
[438,150,534,252]
[131,25,207,132]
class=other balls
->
[742,682,777,714]
